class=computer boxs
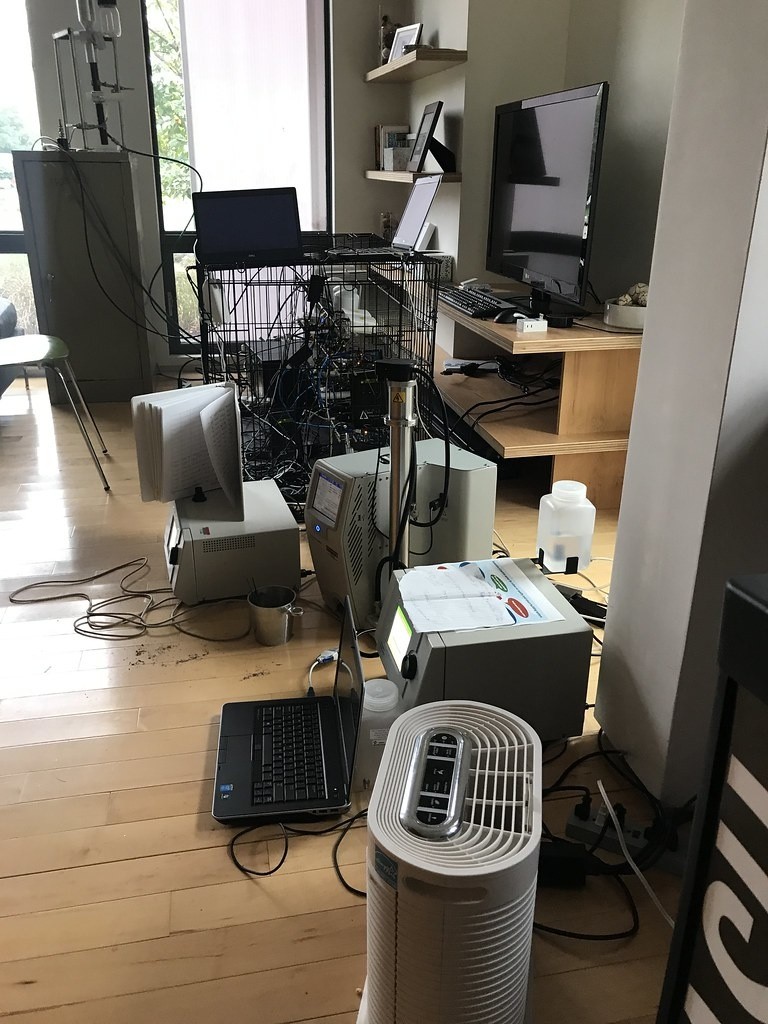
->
[305,438,498,629]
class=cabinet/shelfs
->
[369,262,643,510]
[12,150,158,405]
[362,47,467,287]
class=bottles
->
[536,480,596,575]
[352,679,406,791]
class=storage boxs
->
[385,147,413,171]
[413,253,453,283]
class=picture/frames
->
[387,23,424,61]
[407,101,444,172]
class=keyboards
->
[439,288,518,318]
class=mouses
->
[493,309,533,324]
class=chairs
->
[657,578,768,1024]
[0,334,112,492]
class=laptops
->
[213,593,366,826]
[192,187,317,271]
[327,174,444,261]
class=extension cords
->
[566,808,689,876]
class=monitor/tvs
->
[486,82,611,316]
